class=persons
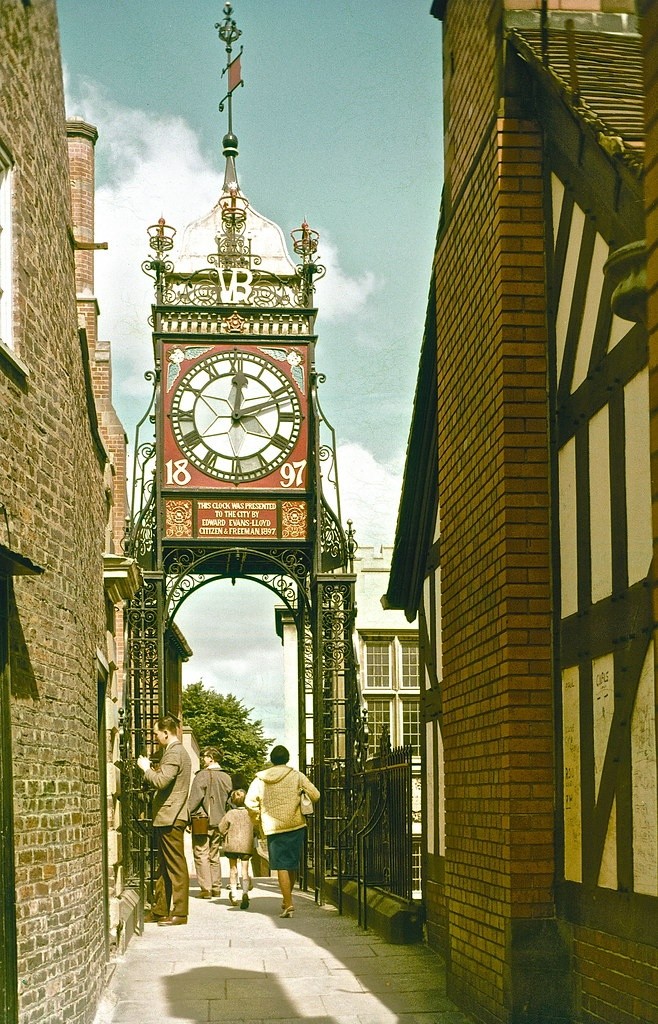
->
[243,745,321,918]
[218,789,256,909]
[188,747,234,899]
[137,715,194,926]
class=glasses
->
[203,754,210,757]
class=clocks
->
[168,349,301,484]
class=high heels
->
[280,906,295,918]
[280,902,286,912]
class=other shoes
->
[230,892,238,905]
[213,890,220,897]
[240,894,249,909]
[195,891,212,899]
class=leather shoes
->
[143,912,168,922]
[157,915,187,925]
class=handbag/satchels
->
[298,771,313,814]
[190,814,210,836]
